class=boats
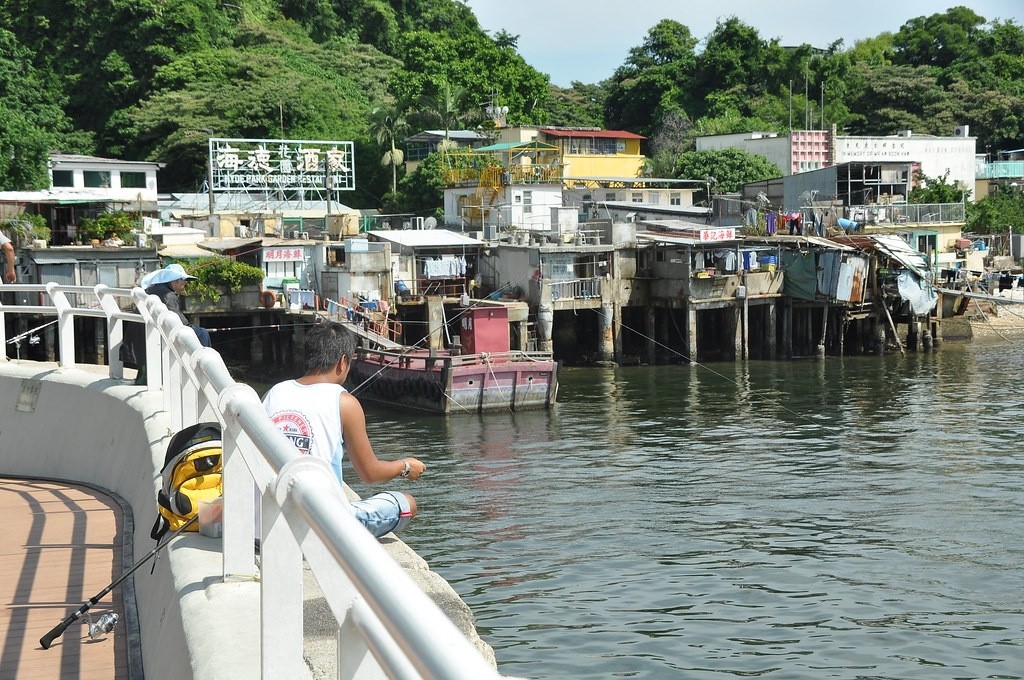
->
[313,293,559,416]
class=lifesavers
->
[260,289,278,309]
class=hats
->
[165,264,198,282]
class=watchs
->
[401,458,411,477]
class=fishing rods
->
[204,321,451,332]
[38,272,526,650]
[6,236,298,345]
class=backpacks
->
[150,421,223,540]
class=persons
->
[520,152,533,180]
[255,322,426,554]
[532,152,541,180]
[0,230,16,362]
[131,264,211,365]
